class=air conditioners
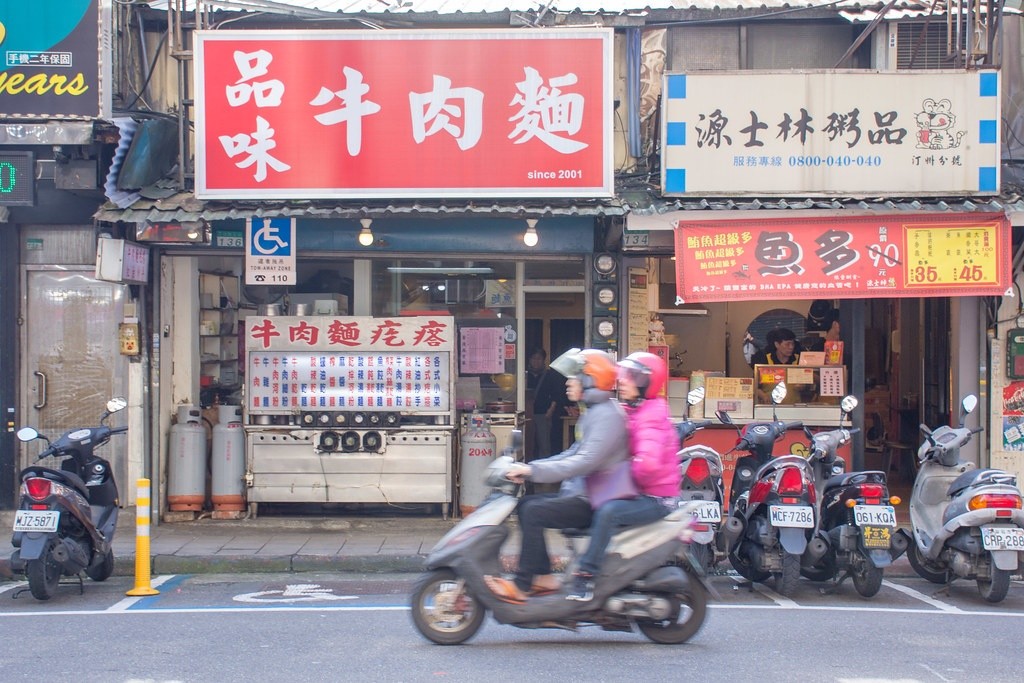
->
[872,20,967,70]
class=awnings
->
[92,179,631,224]
[623,197,1023,232]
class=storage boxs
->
[667,397,689,417]
[668,377,689,398]
[312,299,340,317]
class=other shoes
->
[565,572,595,602]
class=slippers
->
[522,584,560,597]
[482,577,527,605]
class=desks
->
[887,401,919,466]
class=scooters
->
[408,410,717,645]
[905,395,1022,603]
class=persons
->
[759,329,800,365]
[812,309,840,352]
[525,348,558,496]
[742,329,759,365]
[482,348,682,606]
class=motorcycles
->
[674,386,744,580]
[801,394,914,599]
[713,381,828,597]
[11,396,130,602]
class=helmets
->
[549,348,616,390]
[616,352,668,399]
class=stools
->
[881,439,919,484]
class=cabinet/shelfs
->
[198,270,240,388]
